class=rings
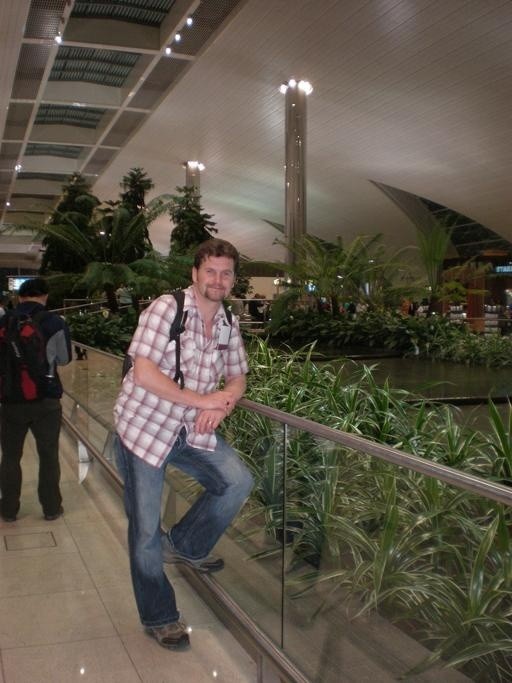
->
[225,400,230,406]
[207,420,213,425]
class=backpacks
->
[121,289,190,381]
[1,311,45,412]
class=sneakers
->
[157,535,224,571]
[45,506,65,520]
[144,620,192,651]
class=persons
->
[109,235,254,653]
[249,290,265,319]
[315,294,430,318]
[0,277,75,522]
[0,291,10,319]
